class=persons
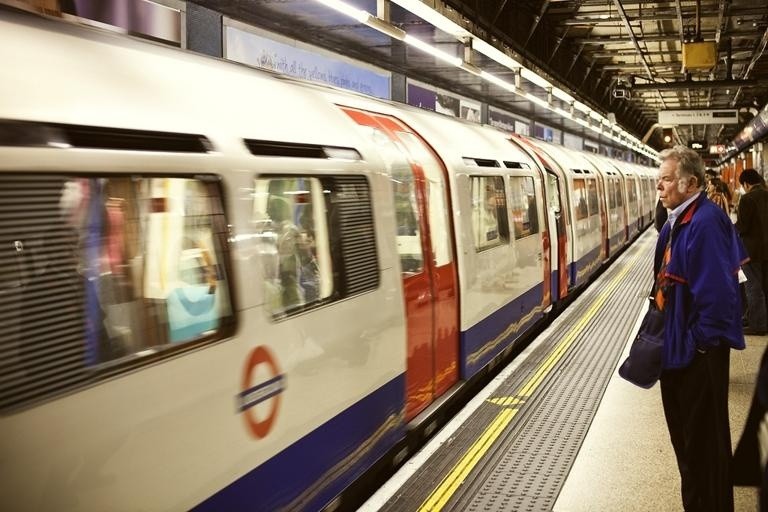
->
[21,222,109,383]
[654,169,768,336]
[618,144,746,512]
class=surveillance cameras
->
[612,88,631,100]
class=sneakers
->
[743,325,767,336]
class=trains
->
[0,0,661,512]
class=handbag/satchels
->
[618,304,672,388]
